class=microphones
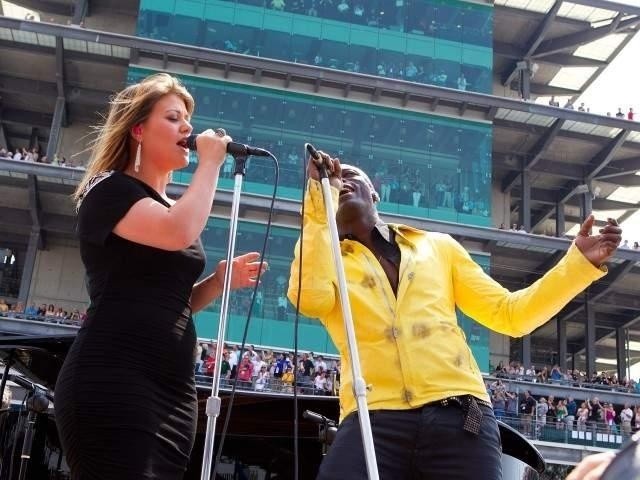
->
[186,134,270,157]
[307,144,333,176]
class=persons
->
[1,145,66,165]
[0,298,86,325]
[54,73,268,480]
[189,1,471,214]
[288,151,622,478]
[195,338,340,396]
[499,223,527,233]
[485,360,640,437]
[25,13,86,29]
[548,95,635,120]
[622,240,639,250]
